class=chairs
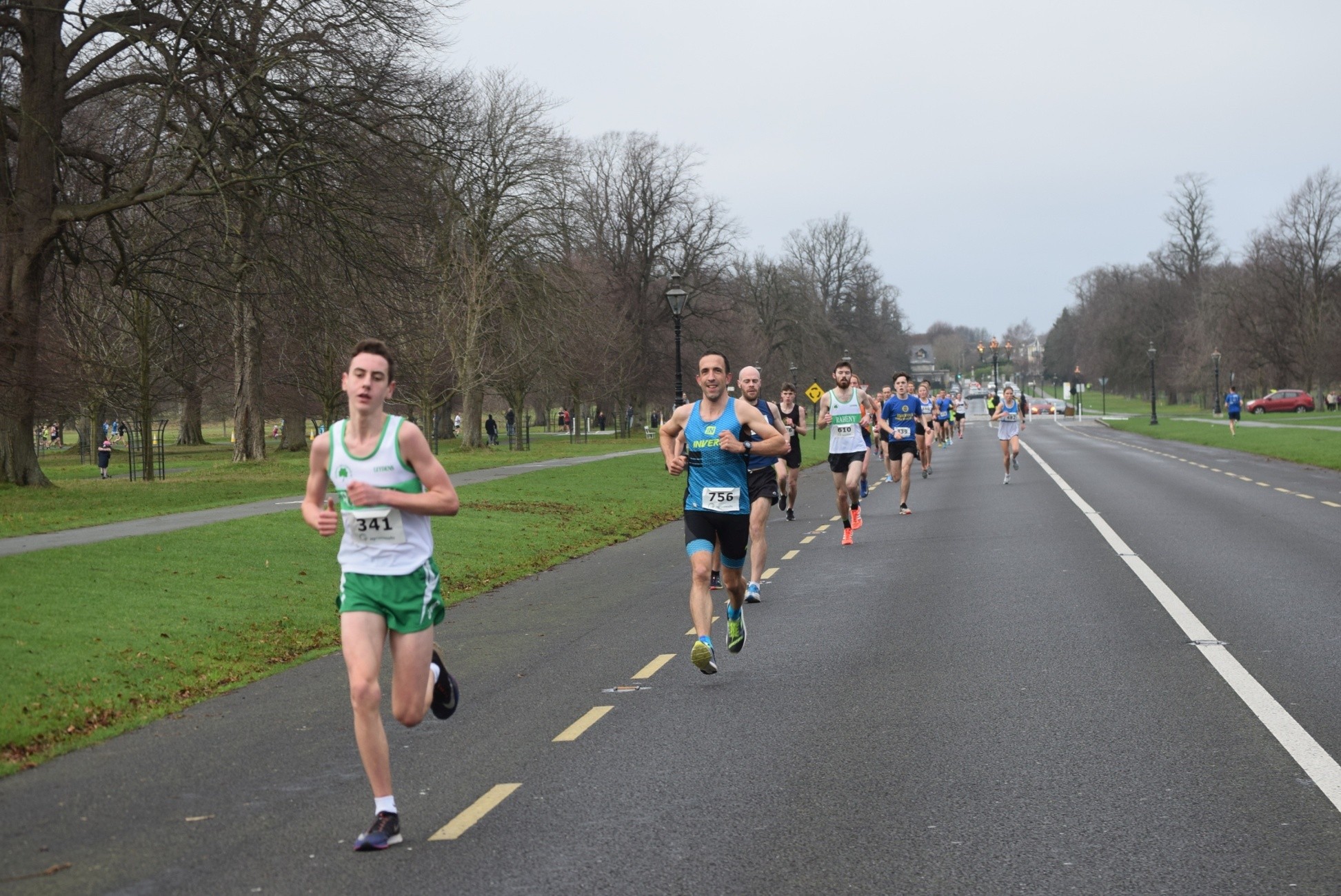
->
[645,426,657,440]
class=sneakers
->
[860,480,869,498]
[691,639,718,675]
[431,649,458,720]
[898,503,912,514]
[885,475,892,482]
[849,504,862,530]
[873,446,884,461]
[725,598,747,654]
[744,584,761,602]
[778,491,795,521]
[842,526,854,544]
[921,465,932,479]
[710,571,723,590]
[353,811,403,849]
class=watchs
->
[742,440,752,455]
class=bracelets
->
[1020,420,1025,423]
[866,413,873,419]
[791,424,796,429]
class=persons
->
[955,393,968,439]
[627,404,635,427]
[274,419,285,437]
[300,338,461,851]
[848,374,881,497]
[273,425,278,439]
[505,408,515,436]
[937,389,956,448]
[817,362,873,545]
[737,365,790,603]
[559,406,564,432]
[599,411,605,430]
[658,348,789,675]
[772,382,807,522]
[879,372,931,514]
[652,410,658,427]
[987,389,1026,418]
[35,422,63,449]
[98,440,112,479]
[915,386,941,478]
[872,380,941,460]
[1325,390,1341,411]
[452,412,462,436]
[946,394,956,445]
[1225,386,1243,435]
[485,414,497,446]
[673,392,691,413]
[103,418,128,447]
[992,386,1025,484]
[710,531,724,589]
[564,408,570,433]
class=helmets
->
[103,441,110,445]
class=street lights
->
[1146,341,1159,425]
[1212,346,1223,415]
[977,336,1014,420]
[666,270,693,470]
[1015,370,1108,415]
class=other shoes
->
[125,444,128,447]
[112,441,115,446]
[1232,433,1236,437]
[1010,453,1019,470]
[116,442,119,445]
[1002,473,1010,484]
[958,431,963,439]
[938,439,953,448]
[100,473,108,478]
[1235,419,1239,428]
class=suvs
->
[1247,389,1314,413]
